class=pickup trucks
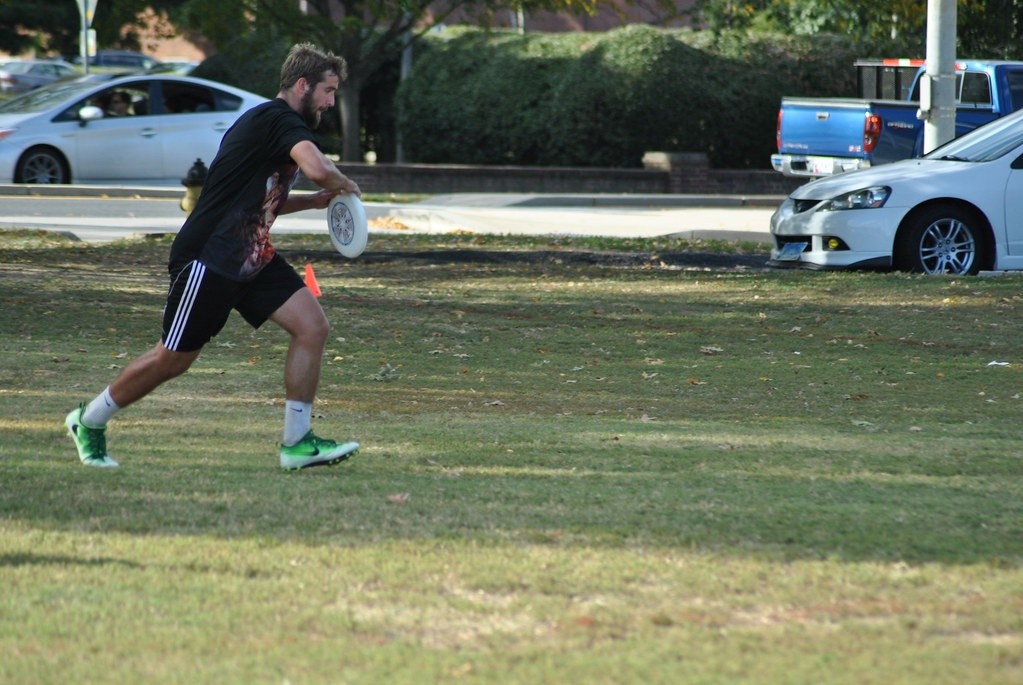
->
[770,59,1023,177]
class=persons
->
[64,40,360,471]
[181,86,215,113]
[85,89,135,117]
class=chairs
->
[134,101,150,114]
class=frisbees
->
[326,191,369,260]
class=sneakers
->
[64,402,119,467]
[280,428,359,473]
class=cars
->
[770,109,1023,279]
[0,50,275,185]
